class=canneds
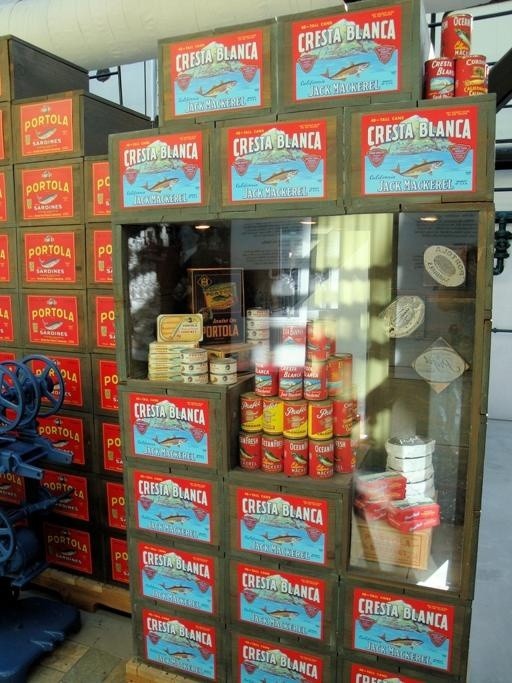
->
[456,53,486,99]
[237,317,359,480]
[423,57,455,100]
[440,13,473,60]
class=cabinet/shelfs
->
[108,199,494,683]
